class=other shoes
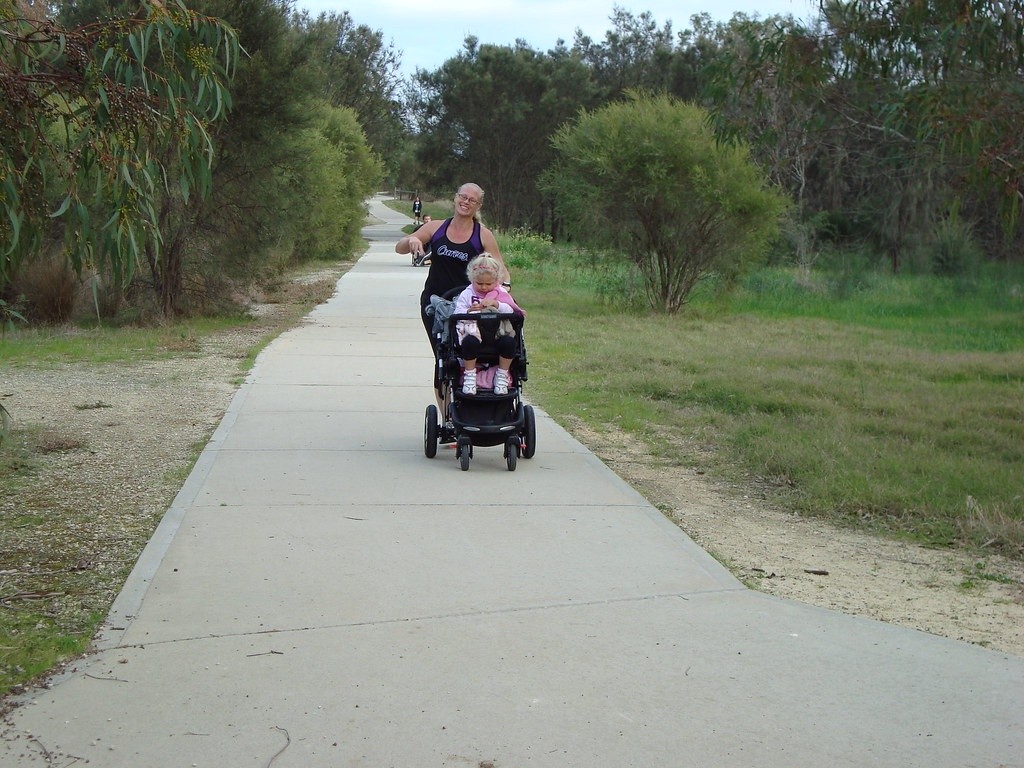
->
[445,417,457,436]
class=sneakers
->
[493,368,509,395]
[462,368,476,395]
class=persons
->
[396,183,516,444]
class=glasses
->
[457,193,480,205]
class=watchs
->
[502,282,511,293]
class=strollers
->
[423,285,535,471]
